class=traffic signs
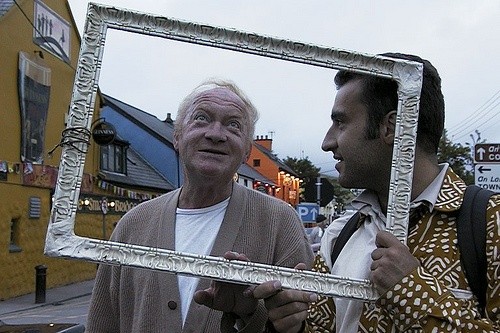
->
[474,143,500,166]
[475,164,500,194]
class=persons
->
[253,51,500,333]
[84,78,314,333]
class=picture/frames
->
[43,1,425,302]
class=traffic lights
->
[297,205,319,224]
